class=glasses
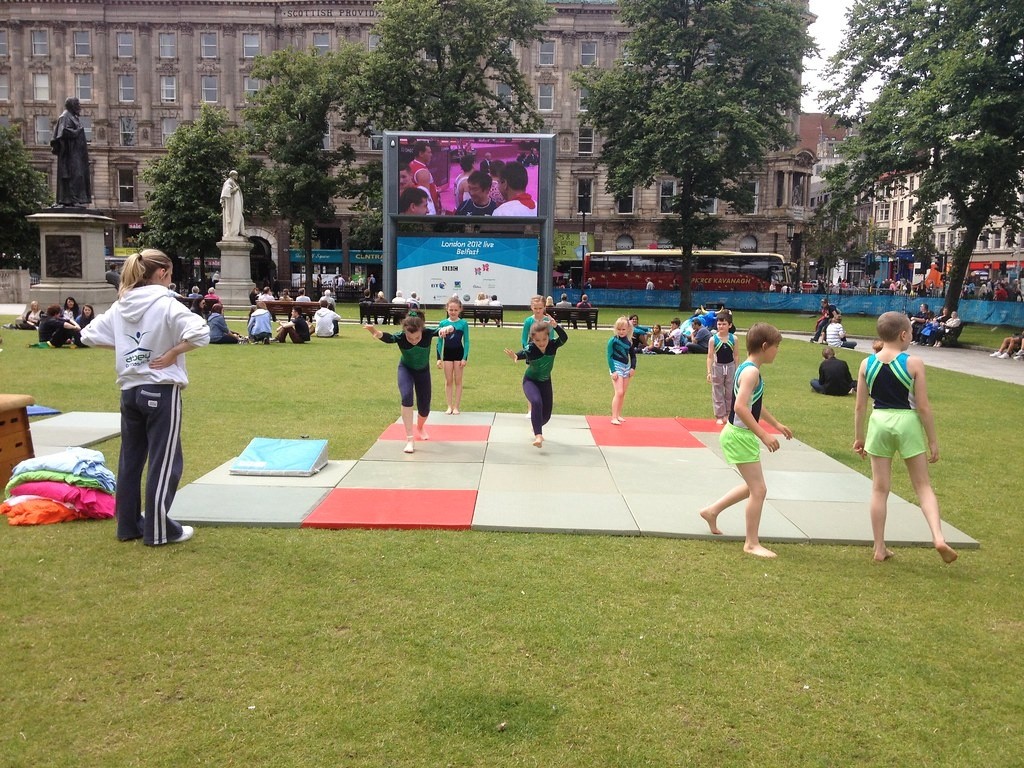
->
[203,302,206,304]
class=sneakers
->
[1013,349,1024,360]
[990,351,1001,357]
[998,353,1009,359]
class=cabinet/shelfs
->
[0,394,37,488]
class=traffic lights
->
[934,254,939,271]
[859,257,865,271]
[1000,262,1004,270]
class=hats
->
[820,299,827,302]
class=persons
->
[398,139,537,214]
[49,96,92,208]
[448,292,593,331]
[810,347,854,396]
[106,261,421,346]
[362,309,455,454]
[219,169,244,242]
[436,296,469,416]
[854,310,957,564]
[706,313,740,426]
[699,322,794,558]
[567,275,1024,360]
[606,316,637,426]
[504,296,568,449]
[79,249,210,547]
[3,297,95,349]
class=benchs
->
[447,306,503,327]
[255,300,320,323]
[546,307,598,330]
[359,302,409,325]
[941,323,966,346]
[176,297,217,318]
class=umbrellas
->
[551,268,565,277]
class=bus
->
[582,249,798,292]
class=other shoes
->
[811,338,827,344]
[270,338,280,342]
[264,337,268,344]
[497,324,500,327]
[74,341,89,348]
[933,341,942,348]
[168,526,195,544]
[909,340,933,346]
